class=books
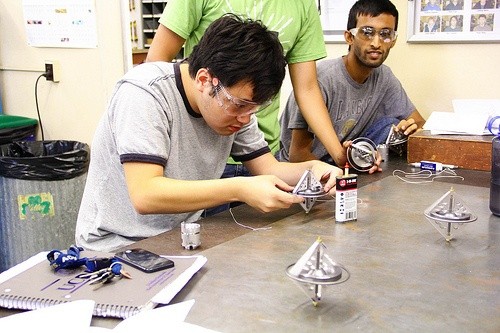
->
[0,253,200,321]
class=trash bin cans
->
[0,115,39,145]
[0,139,89,273]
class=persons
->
[423,0,441,11]
[423,17,437,32]
[473,0,493,9]
[473,14,492,31]
[444,0,462,10]
[443,16,462,32]
[74,12,344,253]
[145,0,383,222]
[274,0,427,168]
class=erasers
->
[420,161,443,172]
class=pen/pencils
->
[408,162,459,169]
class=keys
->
[79,267,130,291]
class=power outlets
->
[43,61,60,84]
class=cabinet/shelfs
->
[141,0,184,62]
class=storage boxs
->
[406,128,500,171]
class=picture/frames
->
[406,0,500,43]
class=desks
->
[0,158,500,333]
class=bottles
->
[488,115,500,218]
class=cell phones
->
[114,248,174,273]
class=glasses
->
[348,26,399,42]
[206,67,273,117]
